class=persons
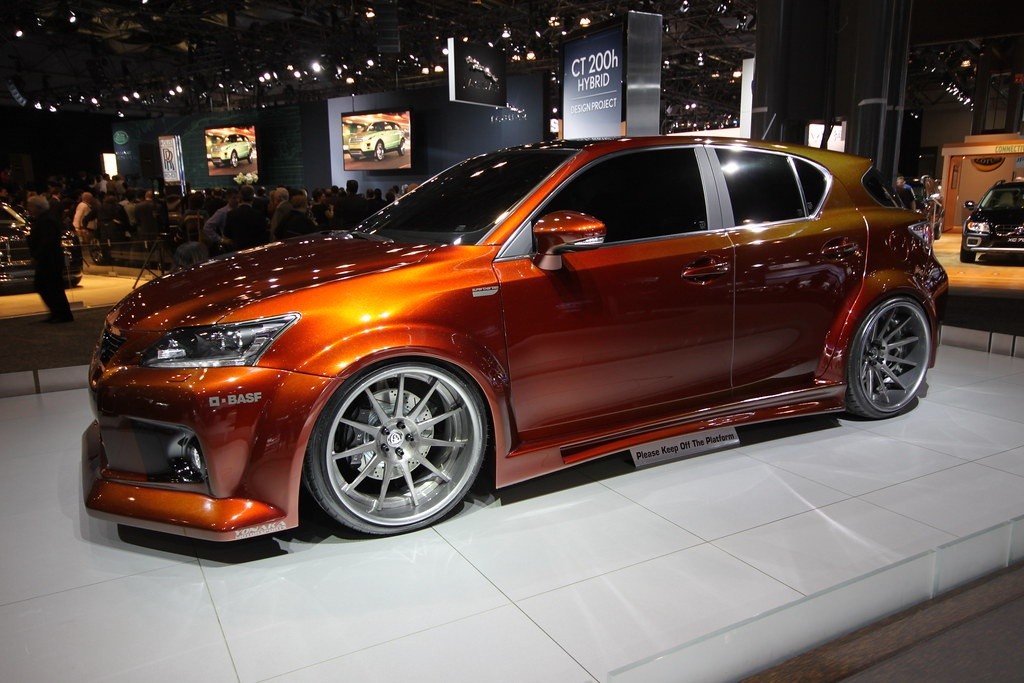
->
[203,188,241,253]
[72,192,97,234]
[274,195,315,241]
[897,176,916,210]
[271,188,293,237]
[25,196,75,323]
[0,166,419,267]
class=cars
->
[88,194,183,266]
[660,112,739,135]
[347,121,405,161]
[78,134,951,543]
[211,134,253,168]
[0,202,82,289]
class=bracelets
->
[221,238,224,243]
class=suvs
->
[960,179,1024,264]
[904,175,945,240]
